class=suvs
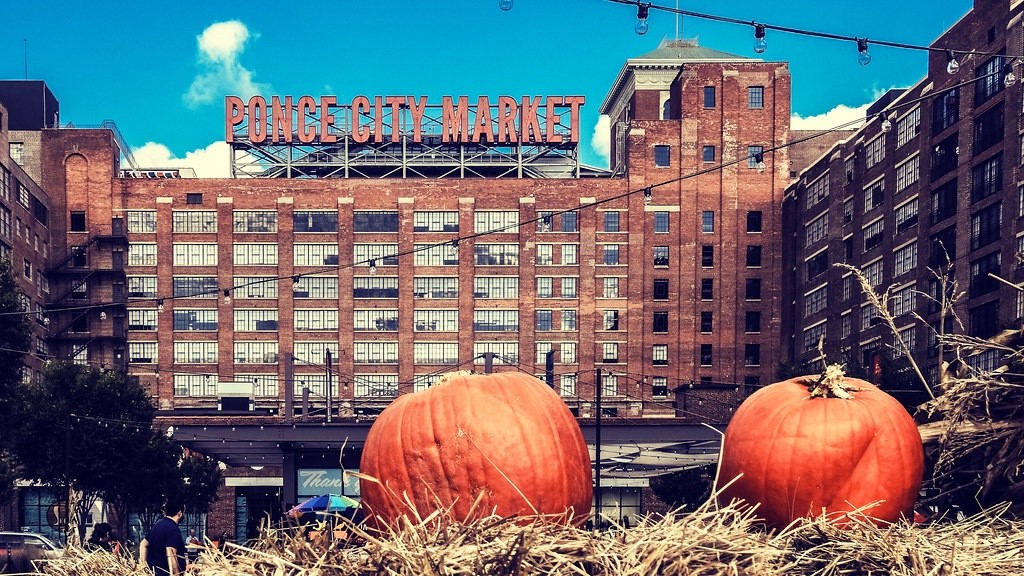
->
[0,531,65,576]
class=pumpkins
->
[715,363,924,536]
[359,369,593,541]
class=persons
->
[84,522,120,563]
[186,529,199,576]
[140,498,186,576]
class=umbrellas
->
[284,493,362,540]
